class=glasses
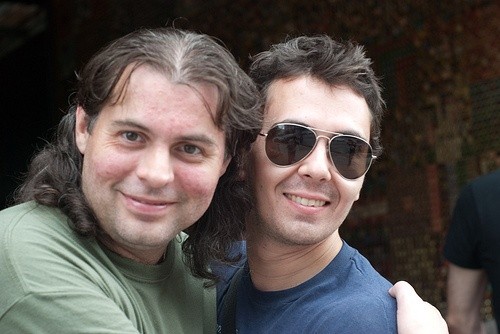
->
[257,122,377,181]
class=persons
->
[441,169,500,334]
[209,34,398,334]
[0,28,449,334]
